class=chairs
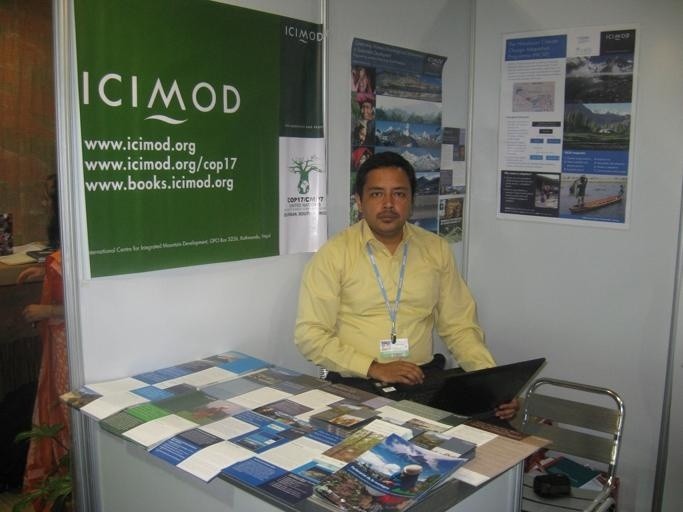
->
[506,375,626,511]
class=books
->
[313,433,467,512]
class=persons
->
[291,149,524,435]
[384,457,422,496]
[349,147,373,172]
[352,92,376,119]
[11,176,78,510]
[353,119,374,149]
[352,65,376,92]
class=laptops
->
[371,357,545,415]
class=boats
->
[567,193,623,215]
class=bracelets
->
[49,304,56,315]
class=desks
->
[57,349,555,512]
